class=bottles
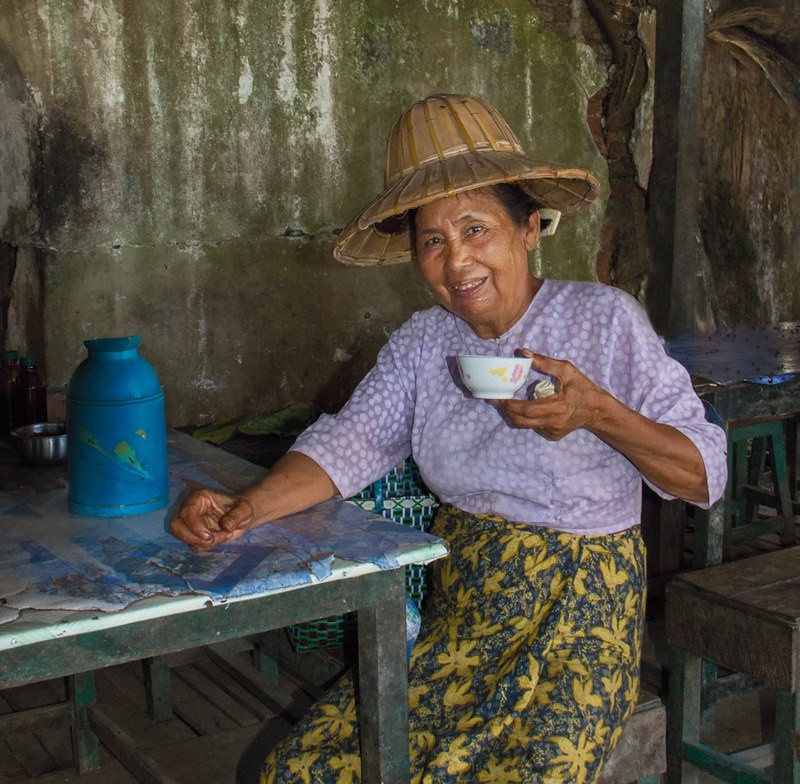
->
[14,358,47,430]
[0,350,20,433]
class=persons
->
[170,91,728,784]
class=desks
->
[0,415,452,784]
[656,320,800,579]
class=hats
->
[333,94,600,267]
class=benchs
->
[663,544,800,784]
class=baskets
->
[288,454,439,654]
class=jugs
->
[66,335,171,517]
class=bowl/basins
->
[11,421,67,467]
[455,354,532,400]
